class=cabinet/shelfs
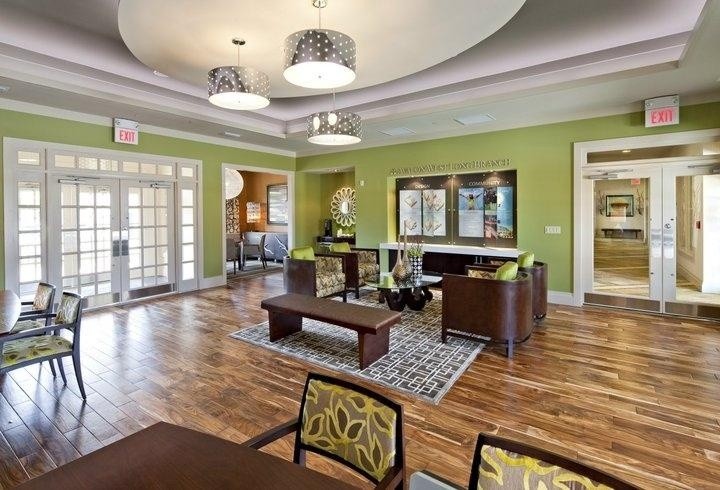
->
[244,232,288,264]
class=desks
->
[10,421,364,489]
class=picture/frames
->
[267,182,289,227]
[606,194,634,219]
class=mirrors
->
[330,187,356,227]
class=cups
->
[384,275,393,287]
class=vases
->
[391,235,408,281]
[402,220,414,279]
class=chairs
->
[441,265,534,361]
[226,233,242,275]
[243,234,269,268]
[466,433,644,490]
[329,248,381,299]
[281,253,348,304]
[237,370,409,490]
[0,290,87,399]
[470,255,549,320]
[9,283,59,378]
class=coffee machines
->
[325,219,332,236]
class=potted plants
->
[407,243,425,278]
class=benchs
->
[258,292,402,367]
[601,227,642,239]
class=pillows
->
[517,250,535,267]
[290,246,317,261]
[495,261,518,281]
[328,242,352,253]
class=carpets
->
[230,280,495,405]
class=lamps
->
[246,201,262,232]
[207,39,272,110]
[225,168,244,200]
[306,88,362,146]
[284,1,357,90]
